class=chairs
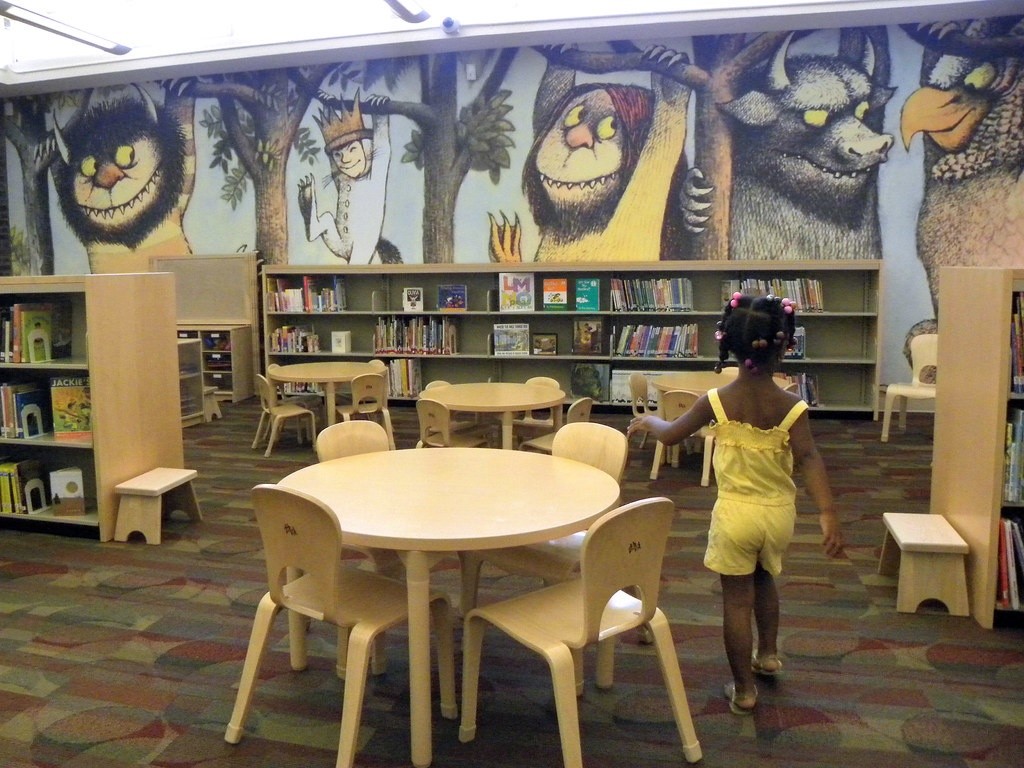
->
[881,333,938,443]
[224,360,795,768]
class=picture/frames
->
[532,334,558,355]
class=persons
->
[627,292,842,715]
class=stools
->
[202,385,222,423]
[878,513,969,617]
[113,467,202,543]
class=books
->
[375,285,468,397]
[1010,291,1024,394]
[998,514,1024,610]
[0,375,92,440]
[176,334,232,417]
[267,275,351,393]
[0,456,85,517]
[494,273,610,401]
[612,278,698,400]
[721,279,824,406]
[0,300,73,363]
[1004,407,1024,502]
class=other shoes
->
[722,681,759,709]
[749,647,781,676]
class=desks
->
[276,449,621,768]
[418,382,566,450]
[652,370,789,467]
[270,362,387,428]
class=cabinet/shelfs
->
[931,264,1024,630]
[268,257,883,423]
[0,272,251,541]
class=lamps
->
[0,1,131,56]
[386,0,430,25]
